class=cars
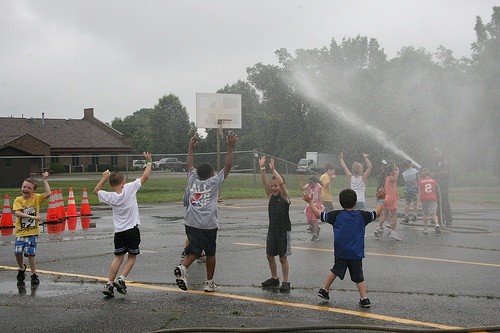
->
[133,157,187,173]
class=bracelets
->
[260,166,265,170]
[379,199,384,203]
[31,216,34,220]
[147,161,152,165]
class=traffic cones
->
[66,187,81,218]
[79,186,94,216]
[0,194,15,228]
[42,189,69,223]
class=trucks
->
[297,151,341,174]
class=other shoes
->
[307,226,312,230]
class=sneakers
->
[174,265,189,291]
[374,231,379,238]
[389,233,404,240]
[16,264,27,281]
[280,281,291,291]
[412,216,417,222]
[422,229,428,234]
[317,288,330,300]
[376,224,384,232]
[359,298,372,308]
[203,279,219,292]
[30,273,40,284]
[402,217,410,223]
[386,223,392,228]
[317,227,321,236]
[113,278,128,295]
[435,225,441,234]
[311,235,320,242]
[102,284,114,296]
[262,277,280,287]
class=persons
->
[418,168,441,234]
[430,148,452,227]
[302,187,386,308]
[258,156,292,292]
[375,156,401,240]
[402,159,419,223]
[13,172,51,285]
[174,133,239,292]
[339,152,373,210]
[296,163,335,241]
[93,152,152,296]
